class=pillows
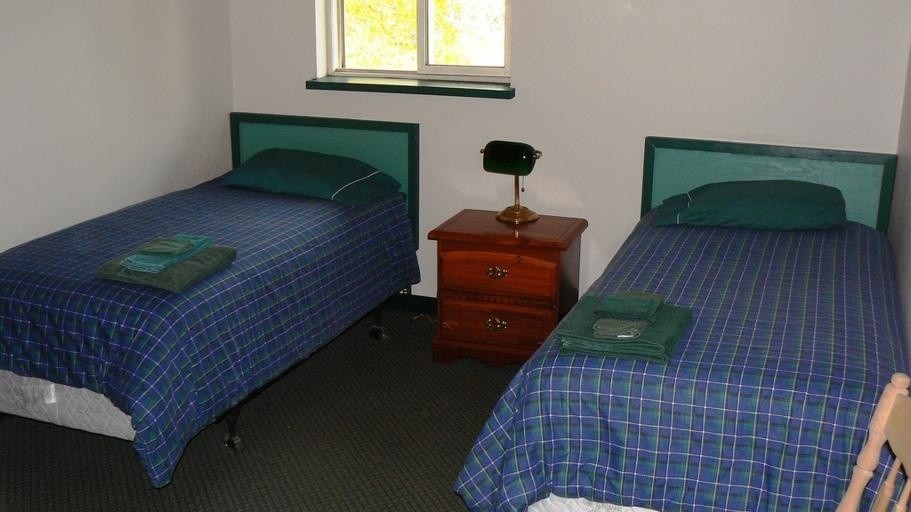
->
[222,147,401,210]
[655,179,848,231]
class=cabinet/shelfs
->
[428,208,589,366]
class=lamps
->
[478,140,542,226]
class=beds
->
[1,112,421,487]
[475,134,910,512]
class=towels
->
[593,291,667,339]
[118,233,215,273]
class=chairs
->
[837,372,910,511]
